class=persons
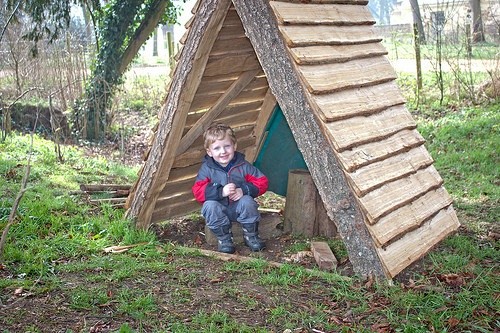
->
[192,123,268,255]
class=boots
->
[210,224,235,253]
[242,221,267,252]
